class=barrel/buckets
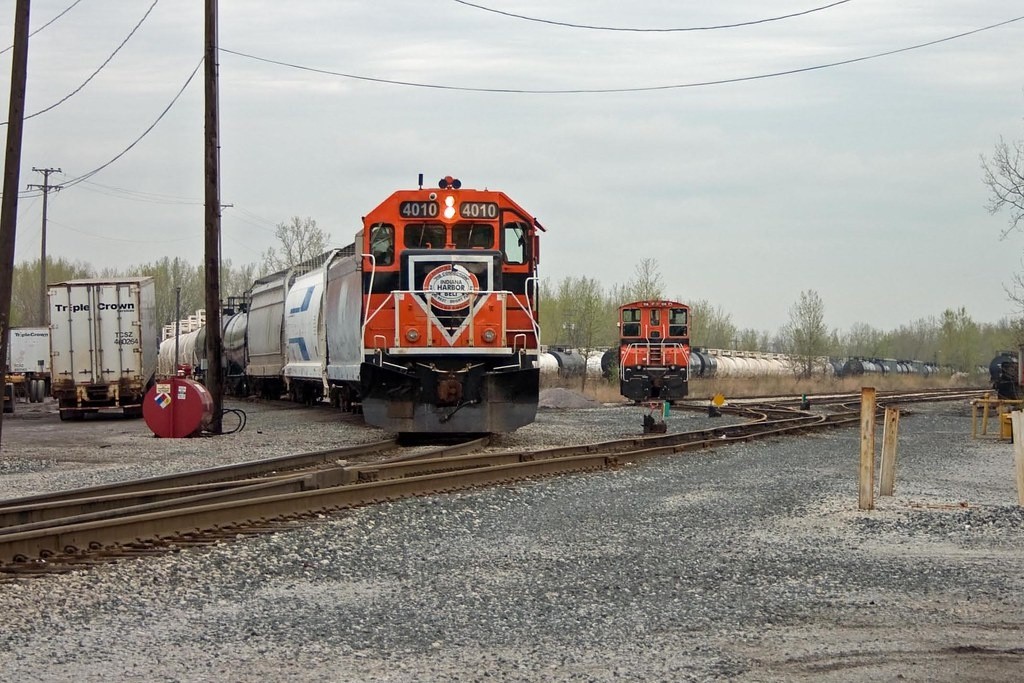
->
[142,378,213,438]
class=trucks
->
[7,326,51,406]
[46,276,161,422]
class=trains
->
[989,349,1023,400]
[159,173,546,436]
[616,300,990,405]
